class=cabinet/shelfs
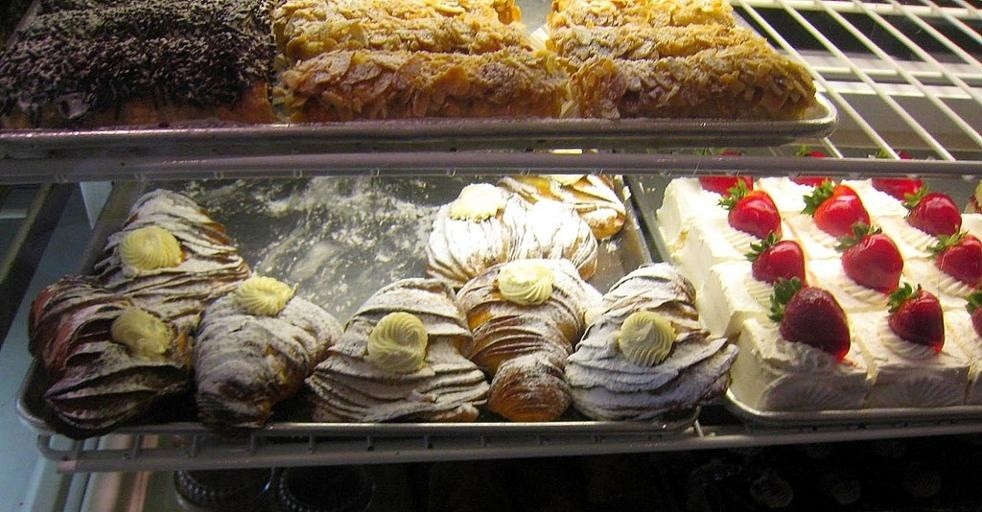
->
[0,0,982,511]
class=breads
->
[1,0,277,133]
[277,1,819,121]
[494,148,627,239]
[456,256,606,422]
[426,174,598,297]
[86,188,253,336]
[305,276,491,424]
[29,275,194,440]
[194,277,345,431]
[566,261,740,422]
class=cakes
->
[658,149,981,411]
